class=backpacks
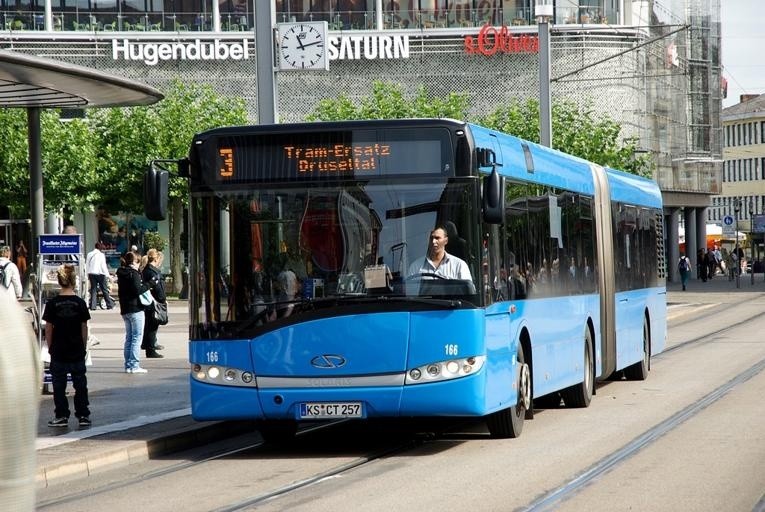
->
[677,255,688,272]
[0,260,14,289]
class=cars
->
[32,259,120,298]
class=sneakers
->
[79,415,92,426]
[124,366,148,374]
[48,416,68,427]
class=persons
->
[249,253,301,326]
[678,253,692,290]
[110,227,127,268]
[0,285,45,512]
[128,229,139,245]
[406,224,476,295]
[85,242,116,312]
[48,225,100,346]
[42,265,92,427]
[116,245,168,374]
[697,243,744,282]
[15,239,27,273]
[509,256,592,288]
[0,246,23,300]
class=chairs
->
[368,15,589,29]
[5,17,239,31]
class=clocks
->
[271,21,329,72]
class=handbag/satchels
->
[152,297,168,325]
[731,253,737,262]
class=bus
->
[148,116,668,441]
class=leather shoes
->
[141,343,164,359]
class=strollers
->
[89,275,114,314]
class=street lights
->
[731,198,741,288]
[748,199,756,285]
